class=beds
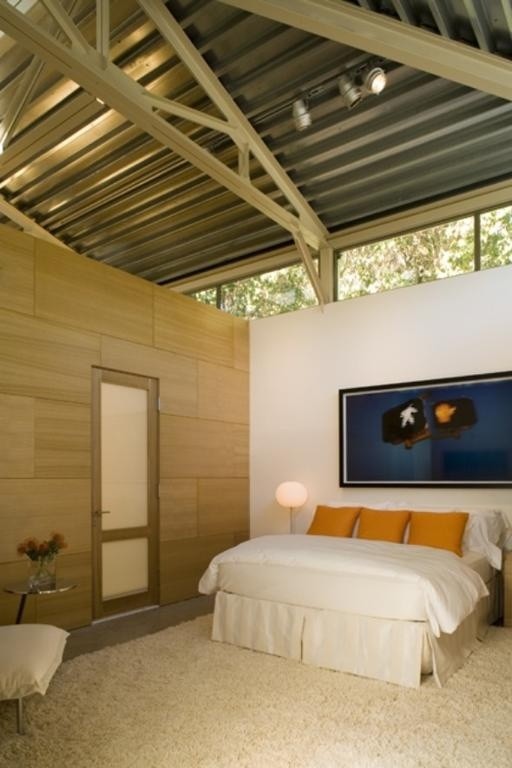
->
[198,504,512,687]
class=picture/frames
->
[339,372,512,489]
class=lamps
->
[275,481,308,533]
[293,54,393,132]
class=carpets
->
[0,613,512,768]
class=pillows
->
[407,512,468,557]
[308,506,360,537]
[357,508,410,544]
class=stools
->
[0,624,70,733]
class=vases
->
[28,557,56,587]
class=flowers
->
[18,532,66,587]
